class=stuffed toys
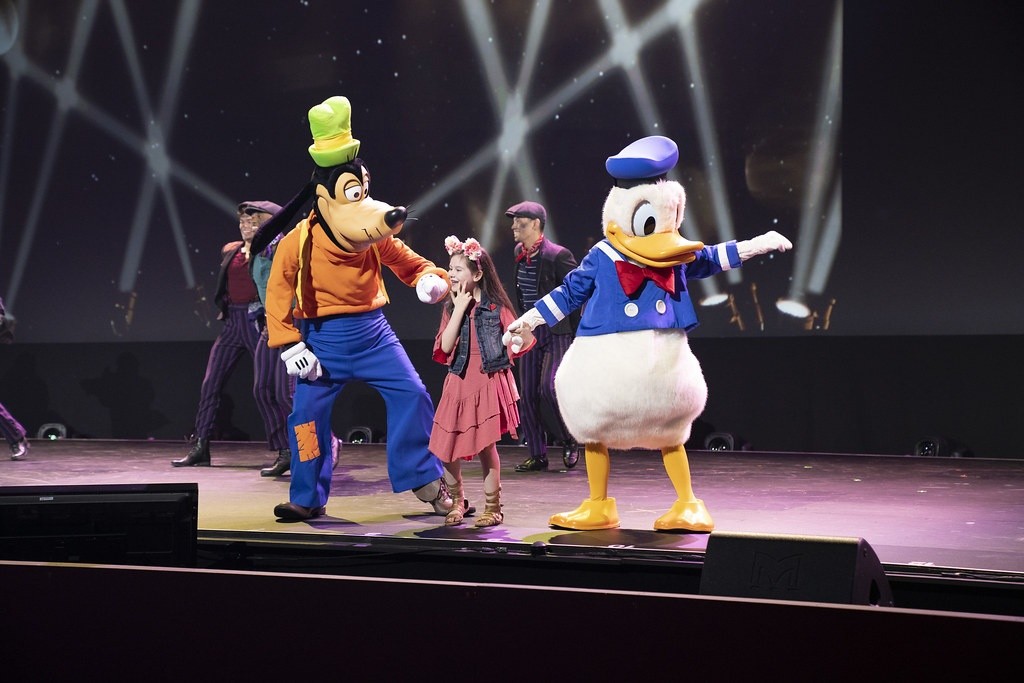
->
[504,135,792,532]
[249,96,475,519]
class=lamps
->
[773,283,810,316]
[697,276,729,306]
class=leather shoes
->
[10,436,30,459]
[260,447,292,476]
[331,433,342,470]
[514,454,548,471]
[563,439,579,468]
[172,435,211,466]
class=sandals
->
[444,477,465,525]
[474,488,504,525]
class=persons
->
[505,201,579,470]
[170,201,342,475]
[427,237,537,527]
[0,298,30,460]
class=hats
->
[237,201,248,212]
[505,200,546,222]
[244,206,267,215]
